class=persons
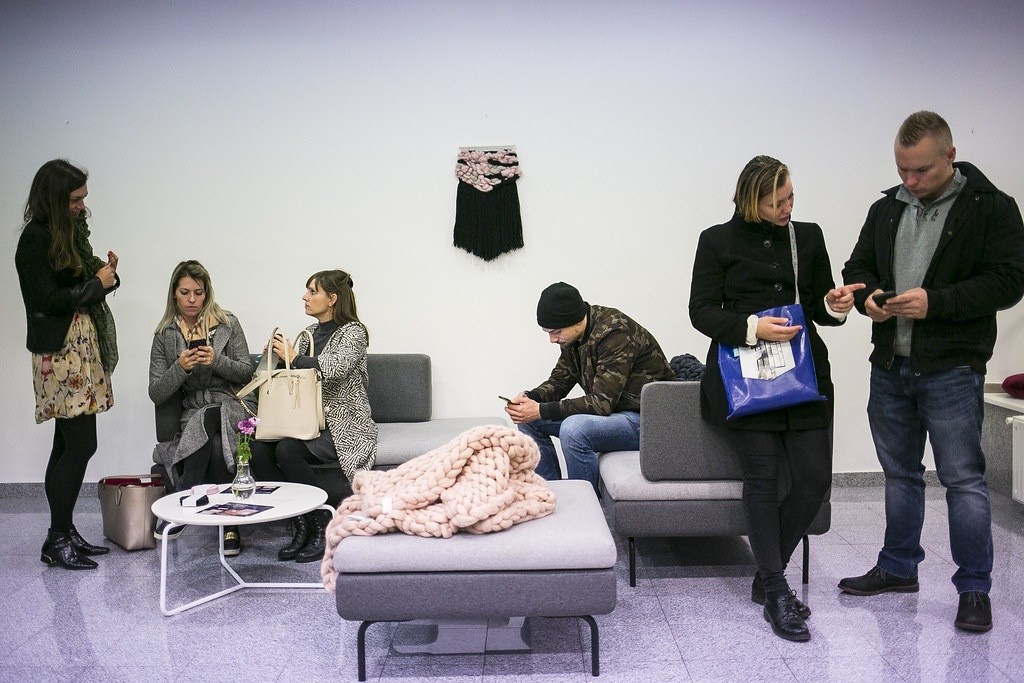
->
[198,506,258,516]
[690,154,865,645]
[246,270,378,562]
[837,109,1024,633]
[505,282,676,492]
[147,260,258,556]
[16,159,121,570]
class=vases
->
[232,464,256,500]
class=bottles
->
[253,334,283,373]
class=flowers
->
[238,418,259,460]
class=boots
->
[279,514,311,560]
[296,509,332,562]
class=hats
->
[538,282,586,329]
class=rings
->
[113,280,117,286]
[204,357,206,361]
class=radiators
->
[1012,414,1024,505]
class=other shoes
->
[154,521,188,540]
[218,529,241,555]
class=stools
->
[320,475,617,682]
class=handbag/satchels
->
[255,327,325,441]
[718,304,827,421]
[98,474,164,550]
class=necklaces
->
[183,318,201,339]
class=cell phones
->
[188,338,207,357]
[872,290,898,309]
[498,395,512,404]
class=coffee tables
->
[151,481,337,616]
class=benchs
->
[598,382,834,587]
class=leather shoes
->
[838,566,919,595]
[764,588,811,640]
[752,572,812,618]
[58,524,110,556]
[955,591,994,631]
[40,528,98,569]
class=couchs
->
[151,351,506,493]
